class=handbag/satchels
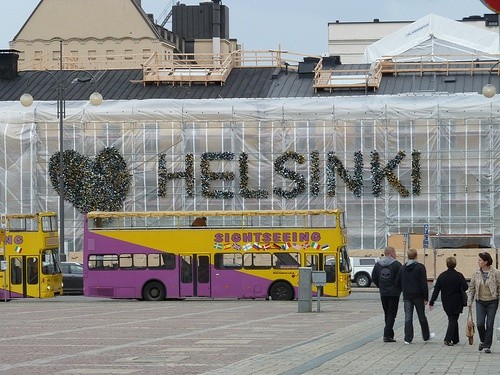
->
[462,290,467,306]
[466,306,475,345]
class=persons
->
[395,248,436,344]
[33,257,38,274]
[191,216,207,226]
[428,257,468,346]
[468,252,500,354]
[371,247,401,342]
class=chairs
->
[113,264,118,269]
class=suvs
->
[325,257,378,288]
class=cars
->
[42,262,84,295]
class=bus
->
[82,210,353,303]
[0,212,64,303]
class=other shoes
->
[444,339,459,346]
[485,347,492,353]
[478,342,484,351]
[426,333,435,340]
[404,341,412,344]
[383,336,396,342]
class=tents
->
[364,14,500,62]
